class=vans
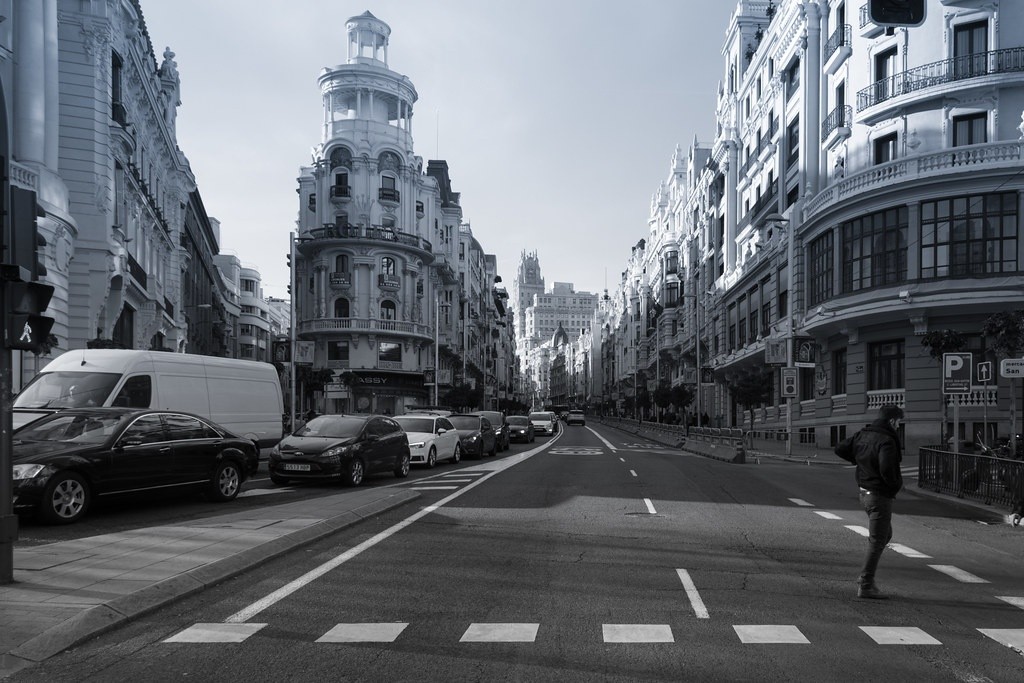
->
[13,349,284,451]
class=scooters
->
[960,431,1024,495]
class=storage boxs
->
[979,483,1006,496]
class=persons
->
[833,403,904,599]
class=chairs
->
[87,422,105,437]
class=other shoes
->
[857,575,864,582]
[858,584,888,598]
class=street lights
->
[435,302,452,407]
[290,230,315,430]
[680,293,701,428]
[628,346,637,428]
[497,357,505,410]
[765,212,793,455]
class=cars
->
[541,412,560,432]
[506,415,535,443]
[269,414,411,488]
[529,412,552,436]
[392,416,461,467]
[12,408,261,523]
[560,410,586,426]
[472,411,510,451]
[446,415,497,457]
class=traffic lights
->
[3,275,56,354]
[13,185,47,280]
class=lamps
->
[899,290,913,303]
[817,306,836,316]
[722,335,766,360]
